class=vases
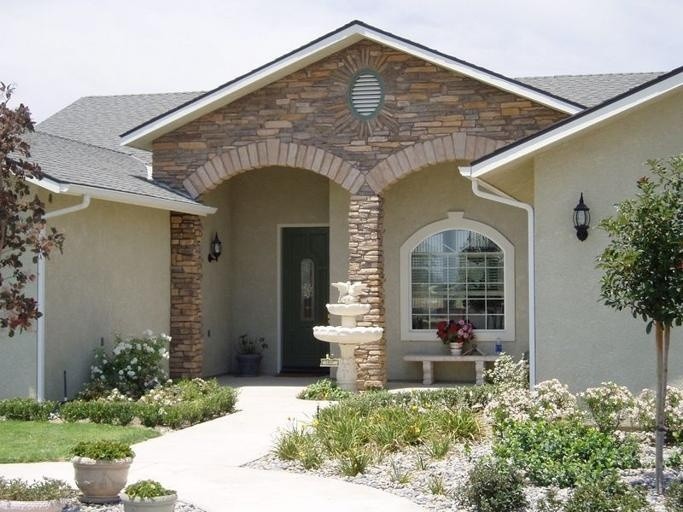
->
[448,342,463,355]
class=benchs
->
[403,352,514,386]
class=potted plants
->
[233,333,268,377]
[0,437,177,512]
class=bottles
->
[495,338,502,355]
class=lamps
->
[571,192,590,241]
[208,231,222,263]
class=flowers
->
[436,319,474,345]
